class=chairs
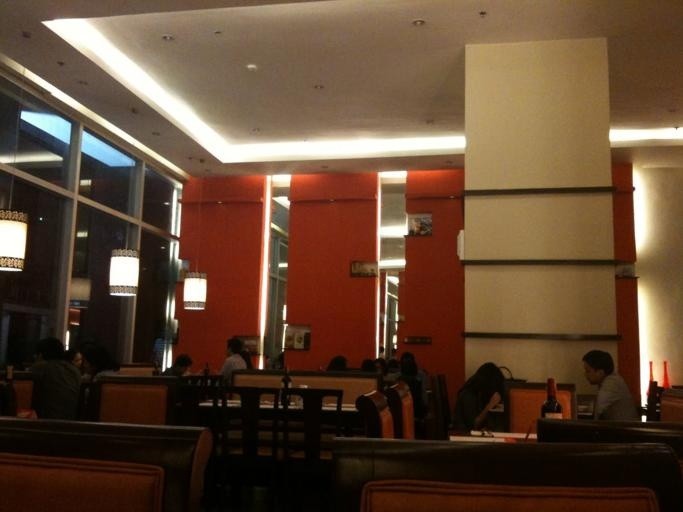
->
[0,362,683,512]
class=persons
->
[449,361,508,431]
[580,350,642,426]
[165,354,192,376]
[221,338,250,377]
[30,338,82,419]
[327,352,427,420]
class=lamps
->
[108,111,141,298]
[183,158,207,313]
[0,32,29,273]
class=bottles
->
[647,381,661,420]
[204,363,209,384]
[541,375,565,421]
[280,365,293,405]
[2,364,17,419]
